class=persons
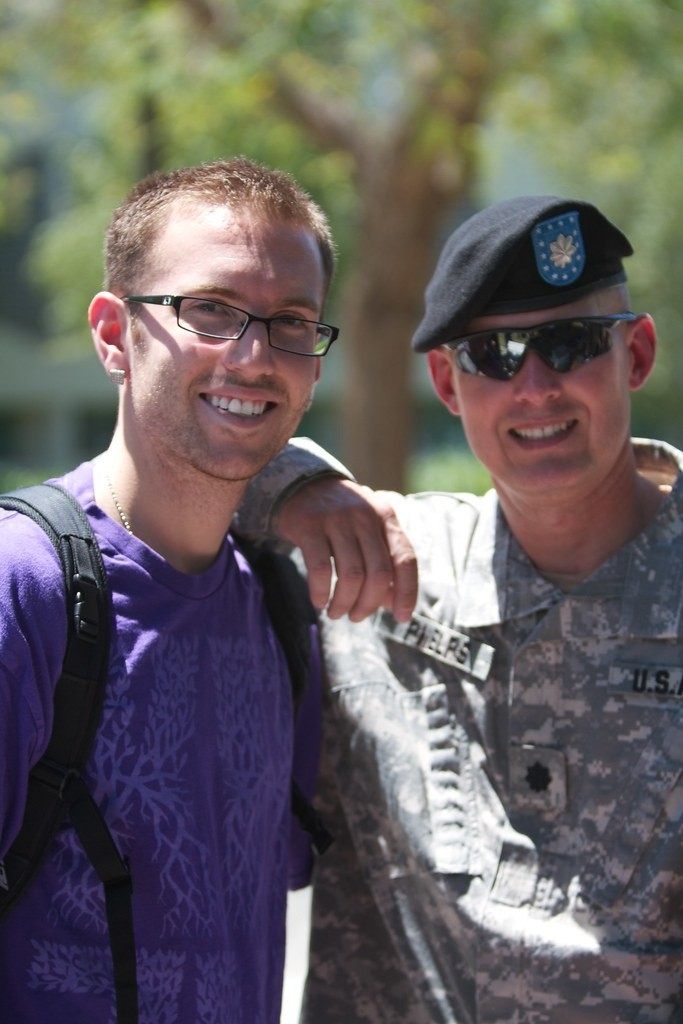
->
[0,158,330,1024]
[227,193,683,1023]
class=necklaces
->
[102,449,132,537]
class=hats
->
[410,195,635,353]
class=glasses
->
[440,311,638,381]
[121,292,341,357]
